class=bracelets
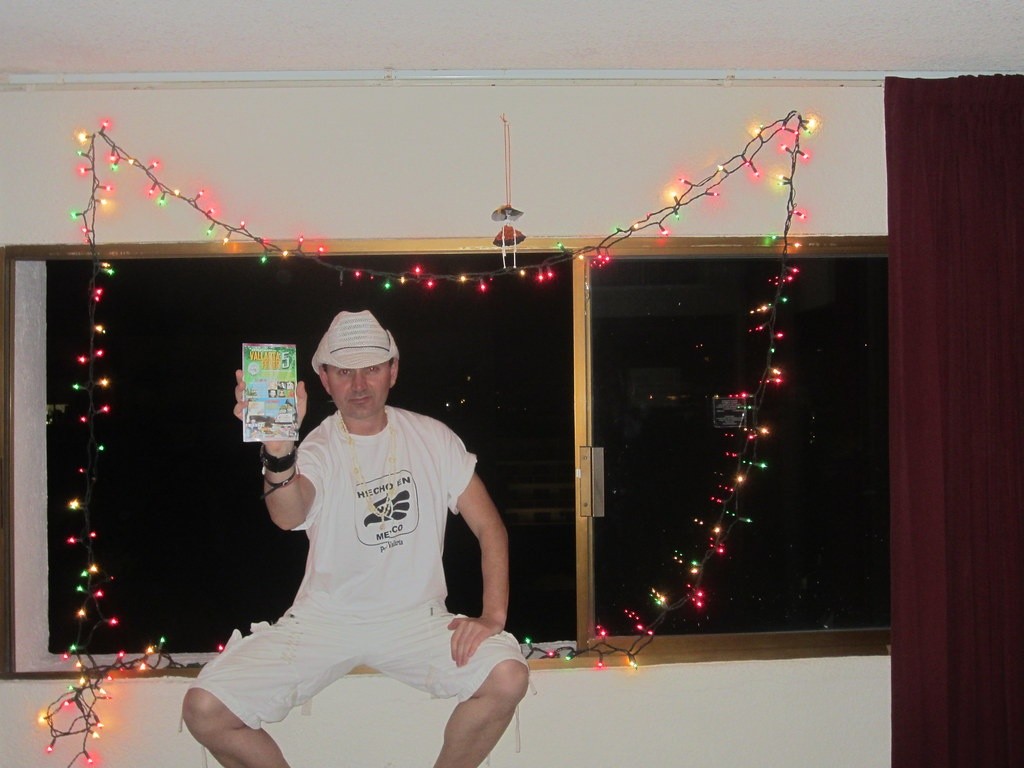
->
[261,463,300,499]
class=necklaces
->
[337,408,398,529]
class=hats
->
[312,310,399,376]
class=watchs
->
[260,444,297,473]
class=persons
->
[182,310,529,767]
